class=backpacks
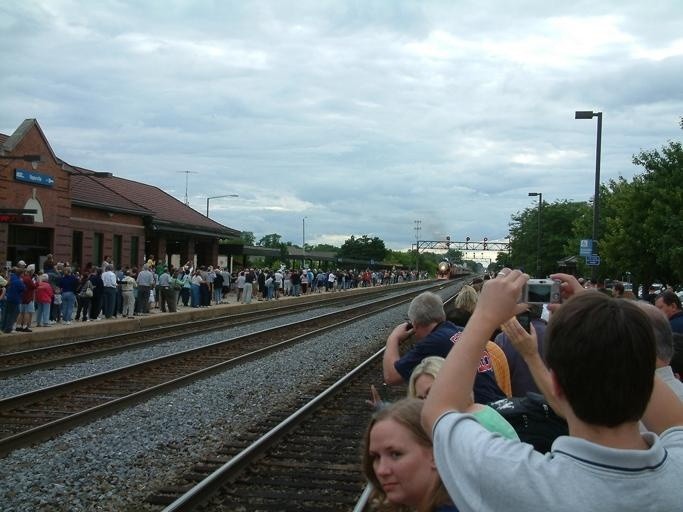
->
[486,390,568,453]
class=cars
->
[604,279,664,301]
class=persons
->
[364,263,683,511]
[0,253,430,336]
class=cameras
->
[522,278,561,305]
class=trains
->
[436,258,471,282]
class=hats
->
[17,260,26,266]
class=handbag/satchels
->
[85,280,93,298]
[174,284,181,290]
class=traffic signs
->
[587,255,600,265]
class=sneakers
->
[16,312,146,332]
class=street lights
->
[178,169,197,206]
[412,218,421,279]
[527,191,543,277]
[574,109,602,290]
[301,216,308,268]
[206,194,238,219]
